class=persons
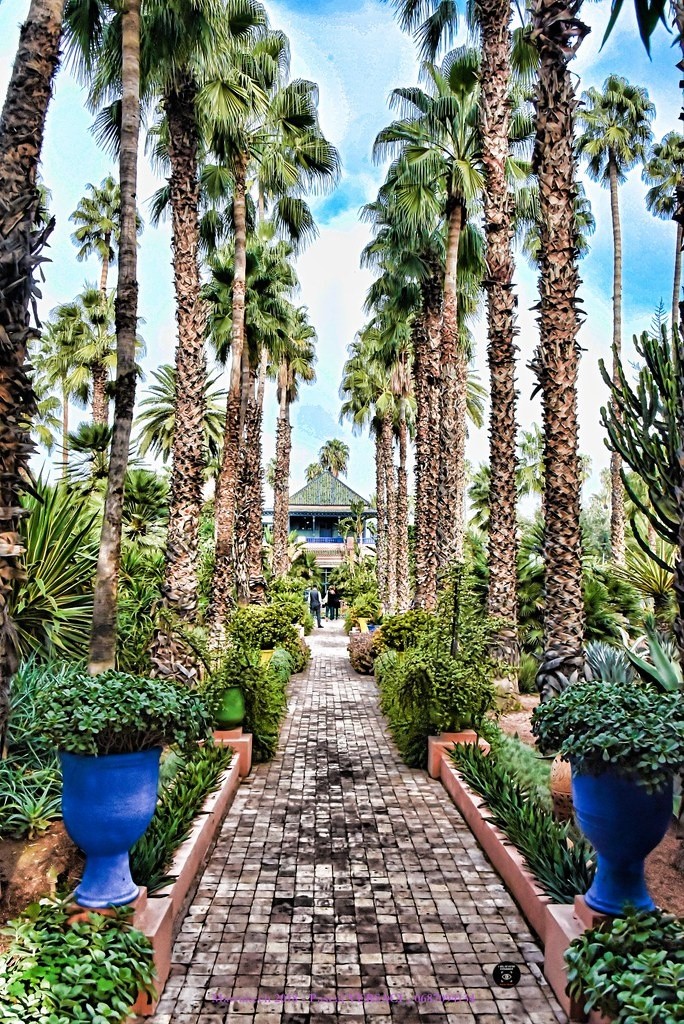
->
[309,585,324,628]
[324,585,340,622]
[303,586,311,611]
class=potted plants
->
[38,672,217,907]
[531,682,682,920]
[200,591,489,736]
